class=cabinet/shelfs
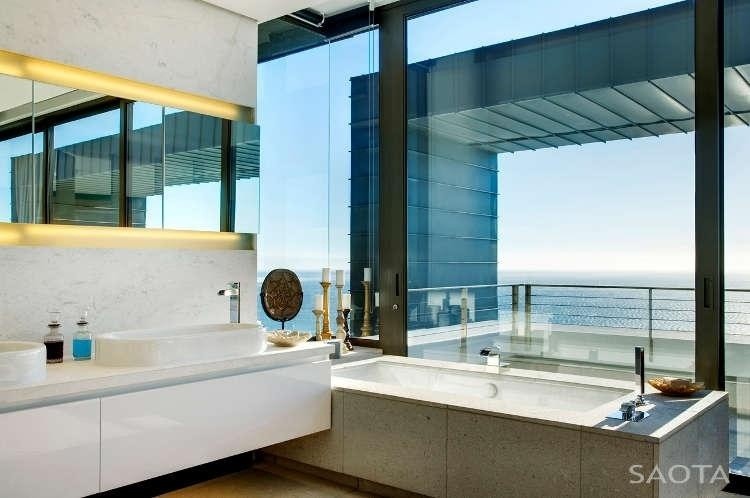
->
[0,354,336,498]
[497,311,550,357]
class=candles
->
[315,268,345,311]
[364,267,371,281]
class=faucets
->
[479,348,500,366]
[217,282,240,323]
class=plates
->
[648,380,706,397]
[266,332,313,348]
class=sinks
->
[95,323,265,365]
[331,361,635,414]
[0,341,46,389]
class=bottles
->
[73,311,93,361]
[44,312,65,364]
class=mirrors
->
[0,74,260,233]
